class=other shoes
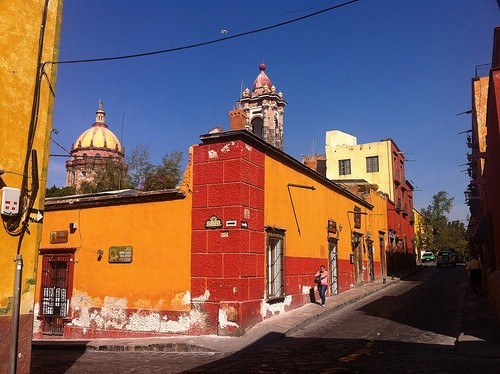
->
[322,304,325,306]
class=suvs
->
[435,250,457,268]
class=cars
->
[421,252,435,263]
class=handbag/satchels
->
[314,276,321,283]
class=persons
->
[315,265,329,308]
[464,256,483,297]
